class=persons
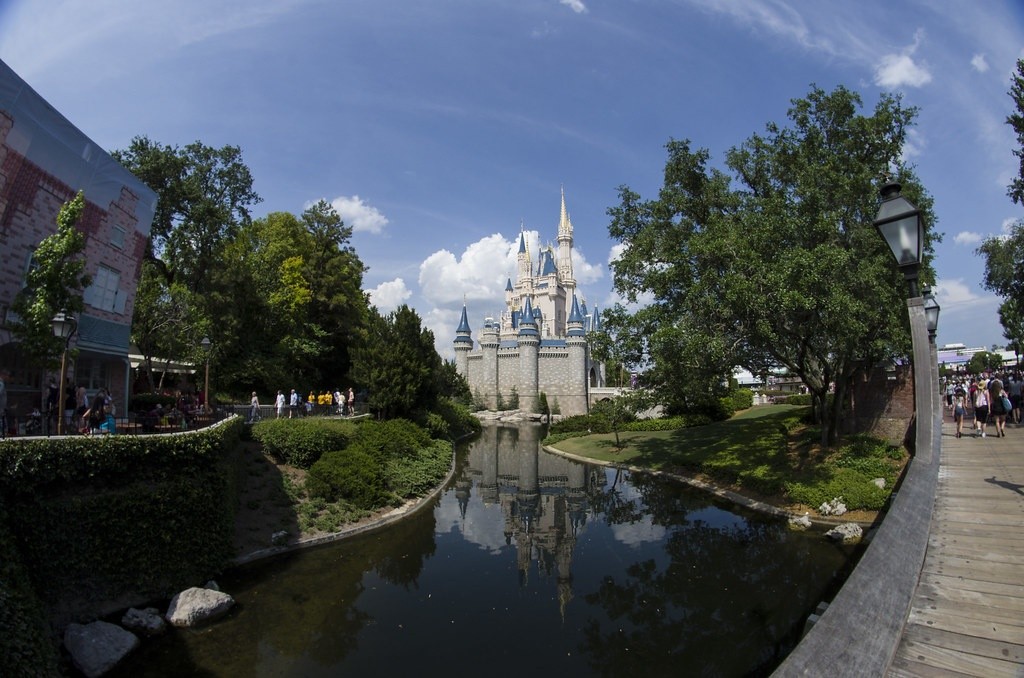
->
[288,389,299,419]
[74,383,89,414]
[308,391,315,403]
[250,391,261,422]
[946,368,1024,423]
[150,404,162,417]
[318,390,333,416]
[334,388,345,417]
[973,380,991,437]
[348,387,355,416]
[32,405,41,417]
[65,378,77,434]
[275,390,285,419]
[951,391,967,438]
[79,387,112,433]
[7,402,17,436]
[45,377,59,434]
[990,381,1008,437]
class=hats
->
[978,381,986,389]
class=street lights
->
[199,332,215,417]
[921,280,939,415]
[49,306,78,436]
[871,181,933,454]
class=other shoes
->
[977,428,981,436]
[998,432,1000,437]
[982,432,985,437]
[957,432,961,438]
[1001,428,1004,437]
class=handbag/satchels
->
[1001,395,1012,411]
